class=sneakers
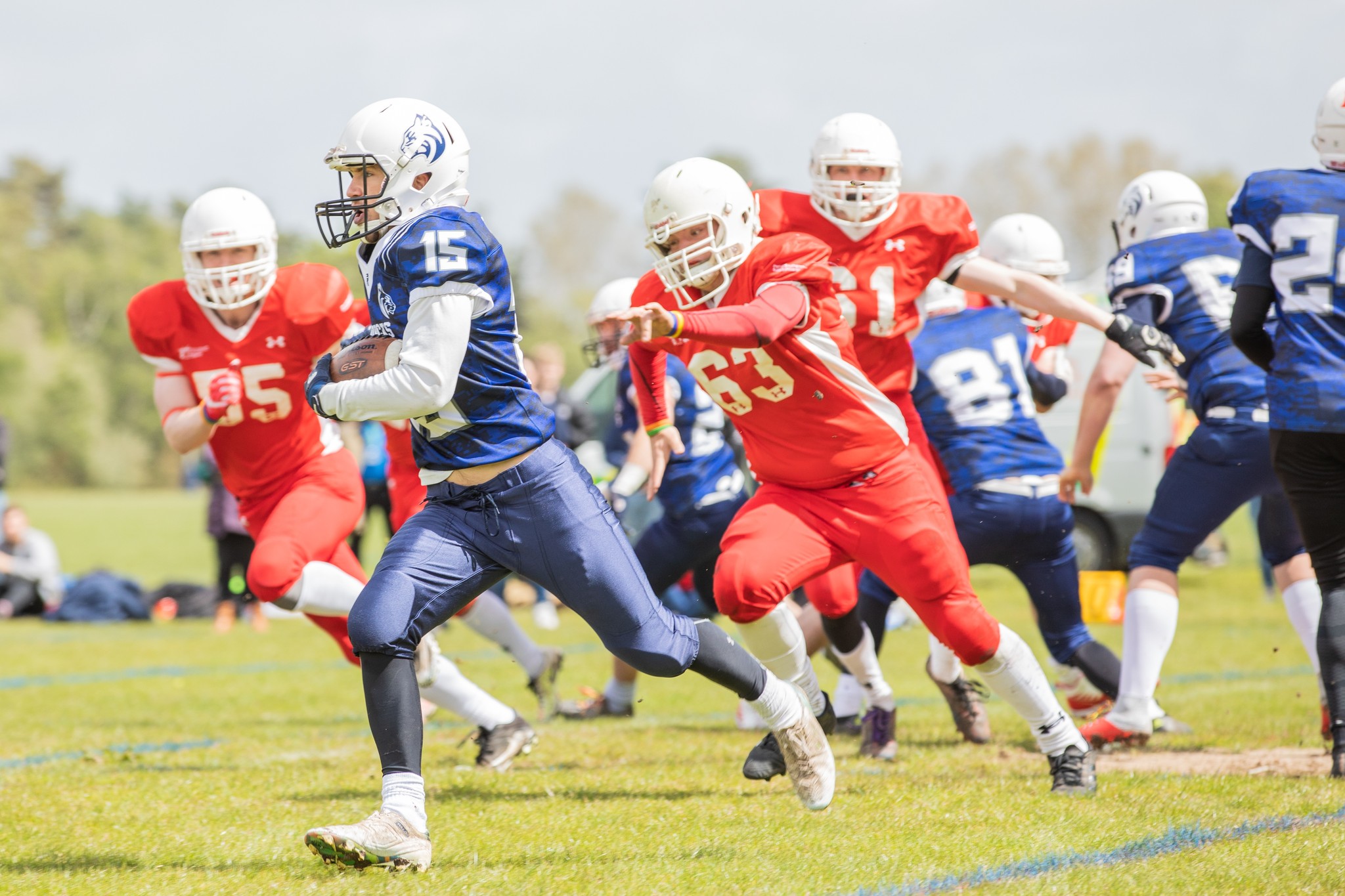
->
[527,650,566,722]
[854,704,900,759]
[1077,700,1151,750]
[303,810,433,875]
[554,685,637,720]
[1057,676,1112,714]
[1152,714,1193,736]
[742,690,836,781]
[1328,753,1345,779]
[1321,704,1334,740]
[831,715,862,735]
[772,678,835,810]
[457,709,538,773]
[1047,736,1099,799]
[924,652,995,744]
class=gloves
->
[339,330,370,349]
[199,363,241,424]
[305,353,333,409]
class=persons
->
[1228,78,1345,776]
[304,96,836,870]
[0,113,1324,797]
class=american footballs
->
[329,334,403,383]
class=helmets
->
[580,274,641,369]
[809,111,900,241]
[180,187,277,309]
[983,213,1072,275]
[1112,170,1210,251]
[316,97,471,249]
[642,157,758,310]
[1312,77,1345,170]
